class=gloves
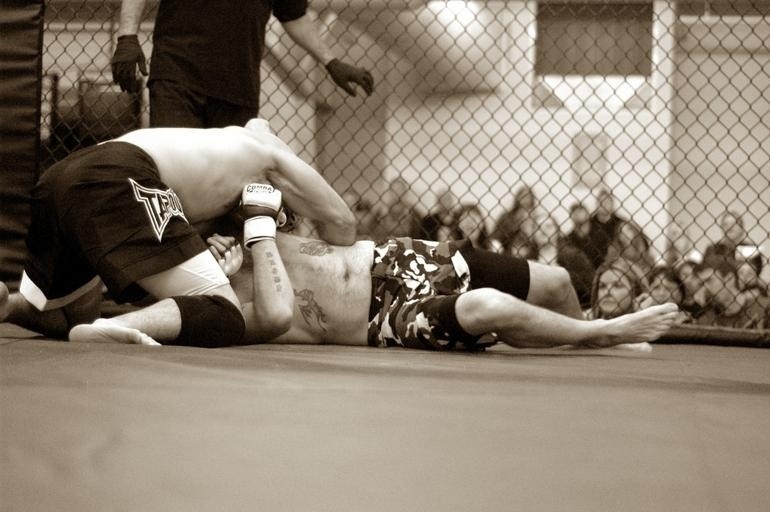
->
[240,179,282,252]
[325,57,373,97]
[110,34,148,94]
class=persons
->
[286,175,769,334]
[0,116,360,345]
[112,1,378,133]
[202,172,681,354]
